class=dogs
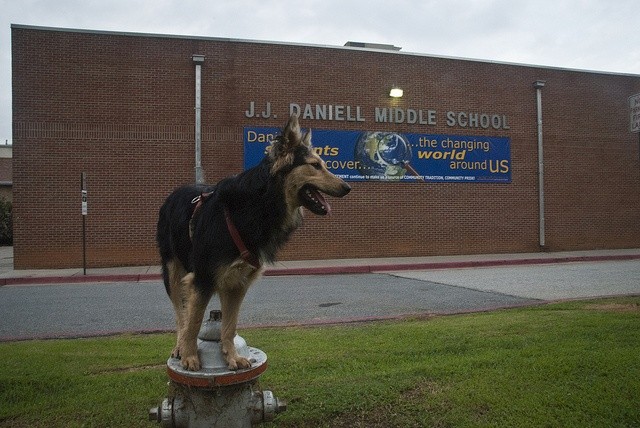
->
[155,112,353,373]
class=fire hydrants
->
[148,309,286,428]
